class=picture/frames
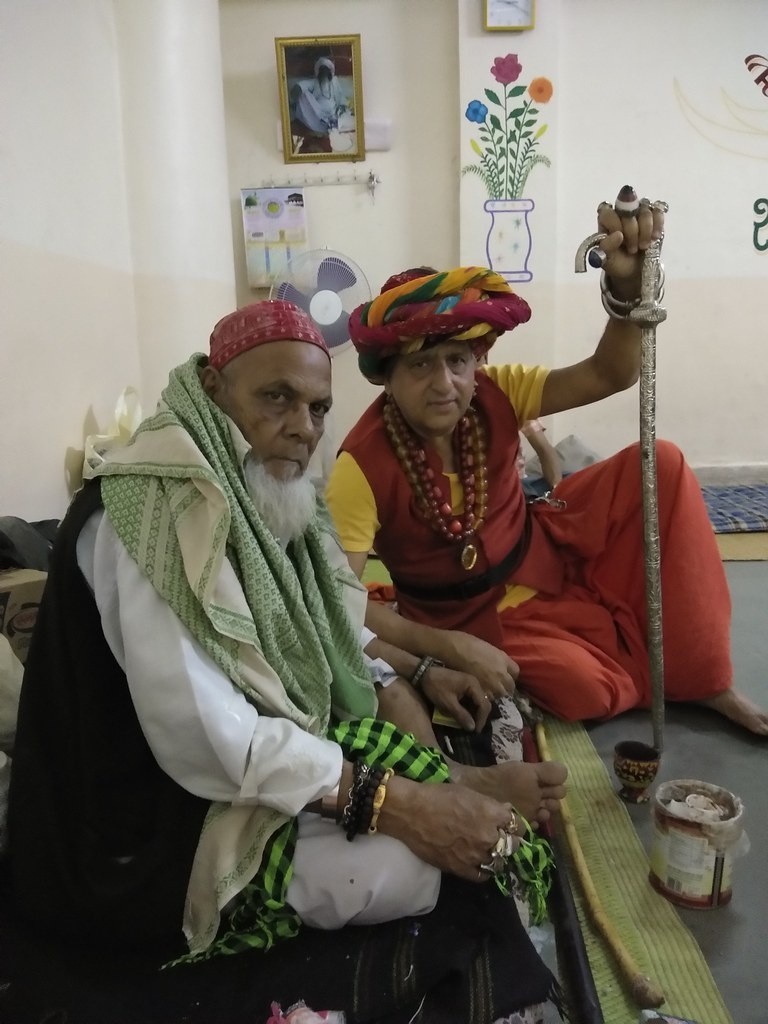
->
[274,34,365,164]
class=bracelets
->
[339,761,394,842]
[411,656,434,688]
[599,262,665,321]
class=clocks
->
[484,0,535,30]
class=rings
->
[475,809,518,881]
[484,696,487,698]
[588,248,606,269]
[614,185,639,217]
[639,197,669,214]
[597,200,612,213]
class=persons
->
[0,298,569,1024]
[321,185,768,736]
[289,57,345,132]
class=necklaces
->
[382,393,487,569]
[318,82,331,100]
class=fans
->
[269,249,372,357]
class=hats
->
[348,266,532,385]
[314,58,335,80]
[209,300,332,371]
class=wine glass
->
[614,741,661,804]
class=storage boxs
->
[0,566,48,664]
